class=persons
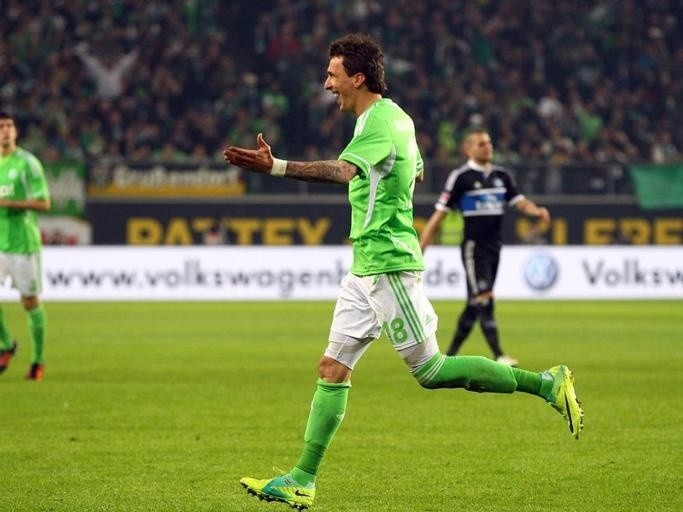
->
[0,1,682,196]
[0,114,52,381]
[221,34,583,509]
[416,126,547,365]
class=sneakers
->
[497,355,518,367]
[240,474,315,510]
[545,365,581,440]
[27,364,43,380]
[0,341,16,375]
